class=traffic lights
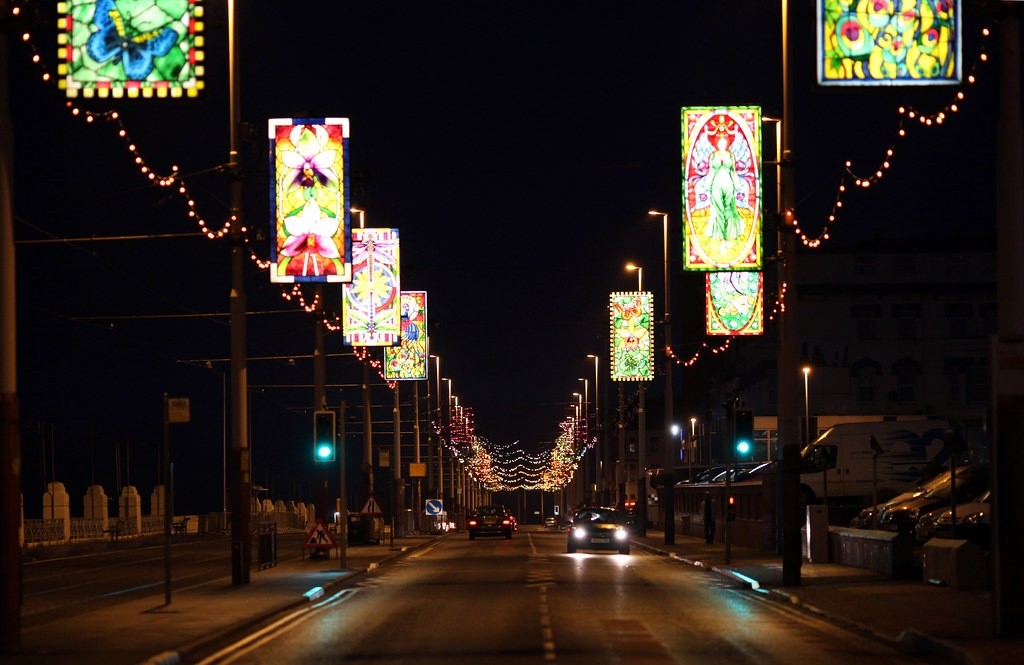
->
[314,410,335,462]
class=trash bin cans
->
[806,504,829,563]
[347,513,386,546]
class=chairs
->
[607,514,615,521]
[583,516,591,520]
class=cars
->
[567,506,631,554]
[545,518,556,528]
[467,504,513,540]
[509,511,517,531]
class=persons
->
[700,492,717,544]
[606,513,616,522]
[723,497,736,538]
[585,513,592,520]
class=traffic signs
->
[425,499,443,515]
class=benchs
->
[173,518,190,535]
[103,519,126,541]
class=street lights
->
[648,209,674,545]
[565,351,603,506]
[625,264,648,538]
[431,353,459,534]
